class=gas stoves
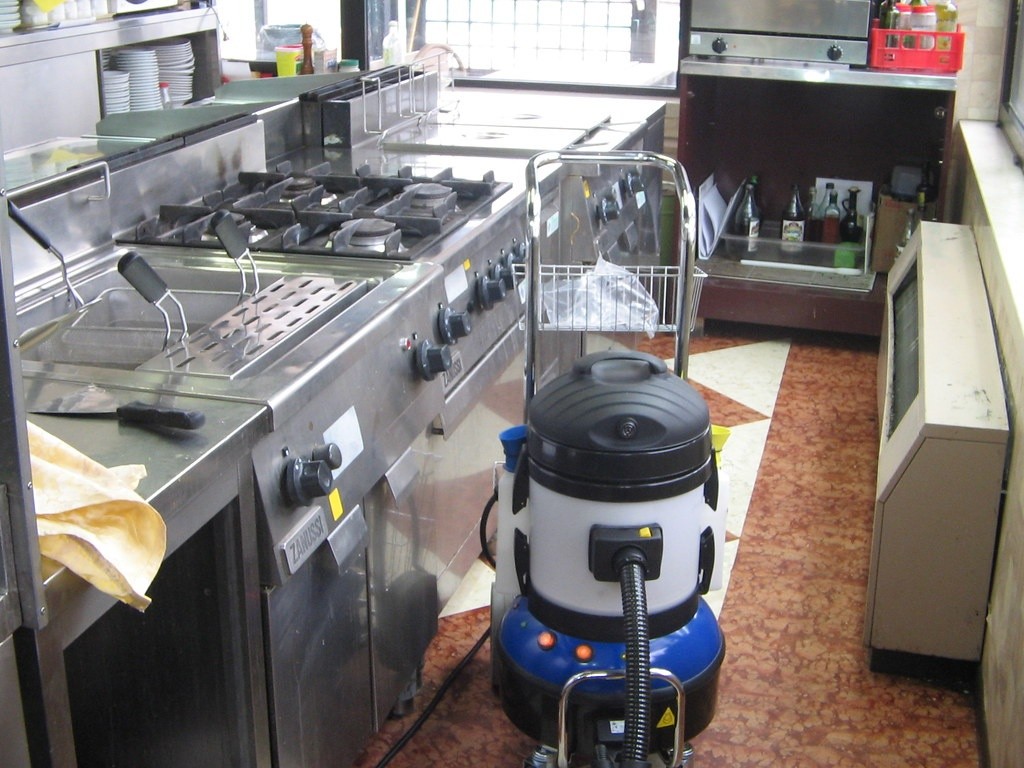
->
[115,170,515,263]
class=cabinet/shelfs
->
[669,63,960,334]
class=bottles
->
[778,164,939,276]
[382,21,401,67]
[728,174,765,261]
[877,0,959,66]
[338,59,360,72]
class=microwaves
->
[107,0,178,15]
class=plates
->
[100,38,196,118]
[0,0,21,35]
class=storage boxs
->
[871,183,938,273]
